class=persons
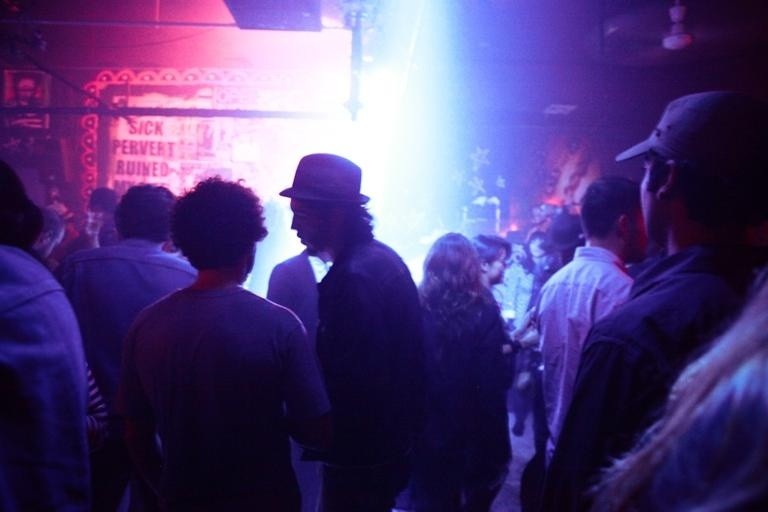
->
[1,73,73,222]
[583,274,768,509]
[277,154,425,510]
[2,159,110,512]
[116,172,333,510]
[34,185,118,270]
[540,86,768,511]
[537,178,648,511]
[56,182,199,511]
[267,247,334,509]
[417,215,586,511]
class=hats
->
[279,152,371,206]
[615,90,767,190]
